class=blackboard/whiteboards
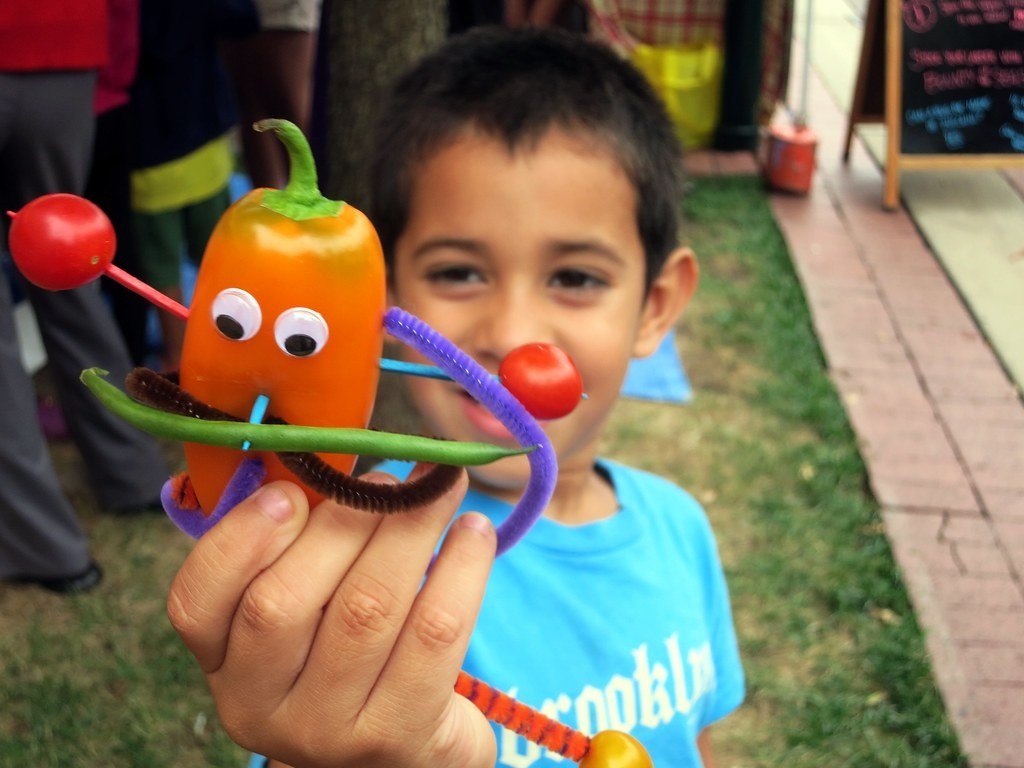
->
[885,1,1024,172]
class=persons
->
[163,22,748,768]
[262,2,454,228]
[0,2,177,594]
[120,2,255,386]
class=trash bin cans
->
[767,104,818,194]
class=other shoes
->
[0,562,100,596]
